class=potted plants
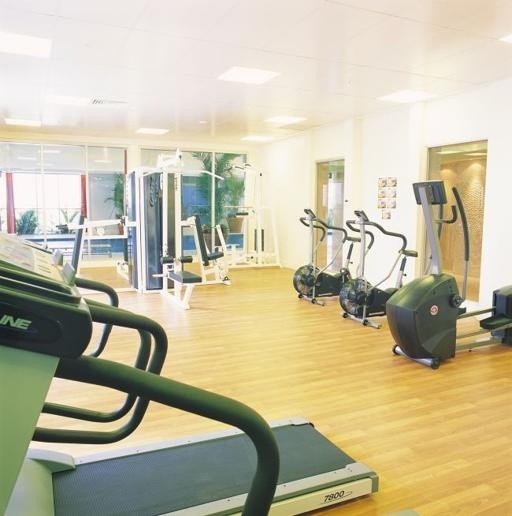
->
[104,174,124,234]
[193,153,239,251]
[222,178,244,234]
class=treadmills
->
[0,231,379,516]
